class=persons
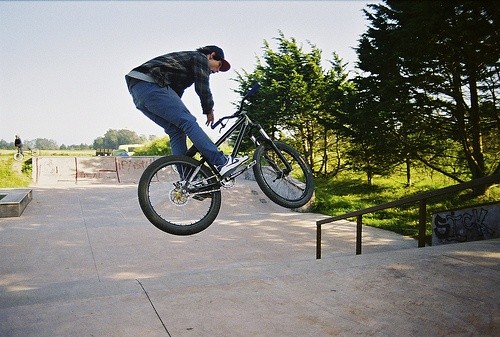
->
[14,134,24,159]
[124,45,249,194]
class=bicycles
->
[12,142,42,161]
[137,79,315,236]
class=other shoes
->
[214,155,250,179]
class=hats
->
[204,44,231,72]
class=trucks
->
[95,147,116,157]
